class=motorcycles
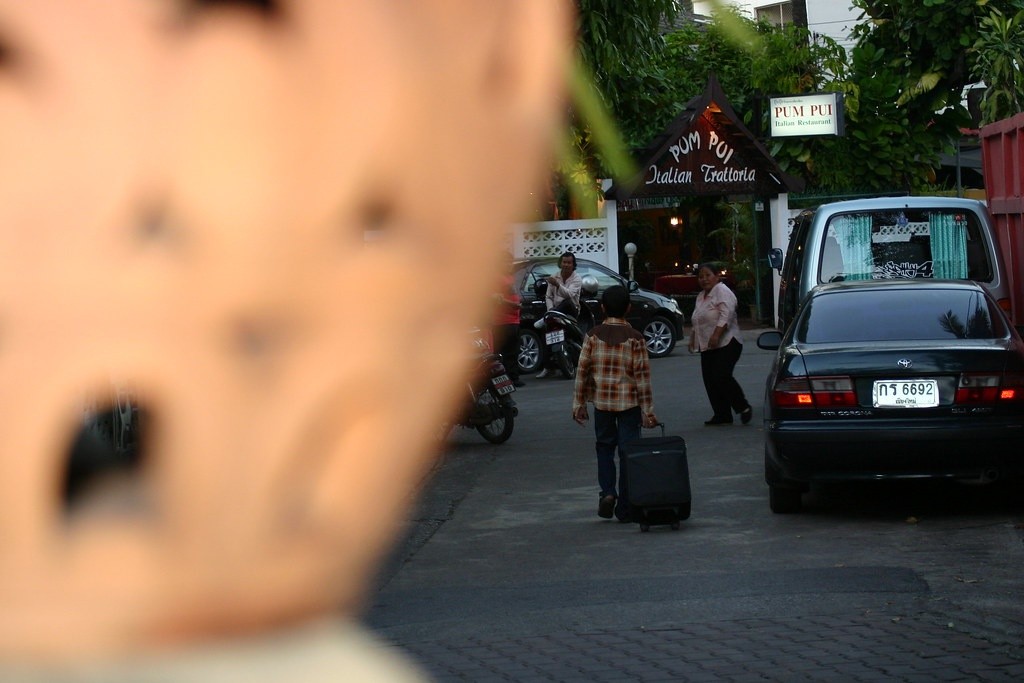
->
[447,337,518,445]
[530,274,600,381]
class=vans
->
[768,194,1015,355]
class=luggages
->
[618,421,692,533]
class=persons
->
[489,251,527,388]
[572,285,657,523]
[534,252,583,378]
[687,263,753,426]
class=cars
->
[754,277,1024,514]
[512,255,686,359]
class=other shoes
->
[513,380,524,387]
[704,414,733,425]
[741,405,751,426]
[598,495,614,518]
[536,368,553,378]
[534,317,547,329]
